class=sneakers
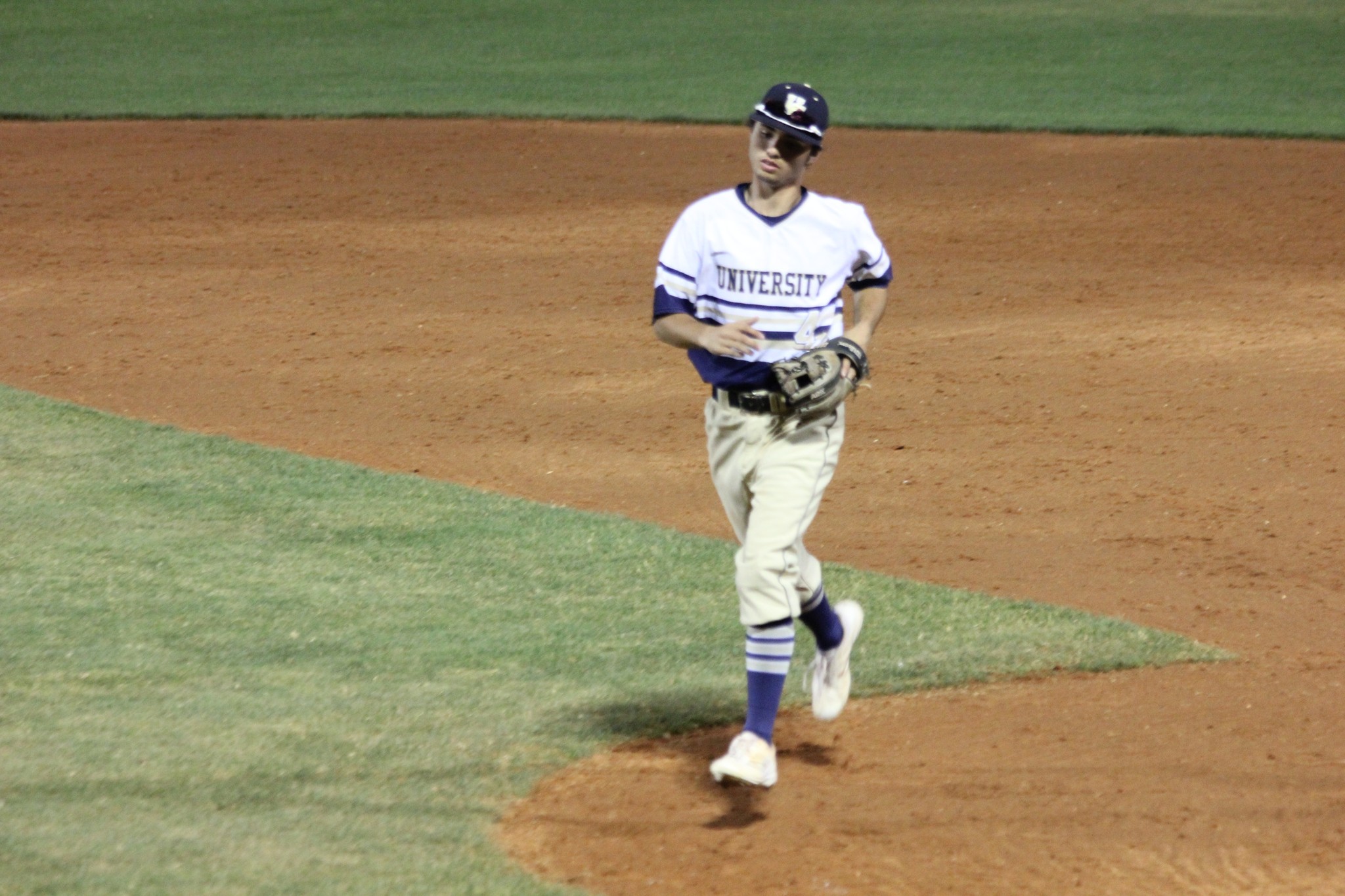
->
[710,732,778,791]
[810,599,865,720]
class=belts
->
[712,386,792,413]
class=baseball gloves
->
[763,335,878,444]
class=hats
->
[748,82,830,149]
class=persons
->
[649,81,895,794]
